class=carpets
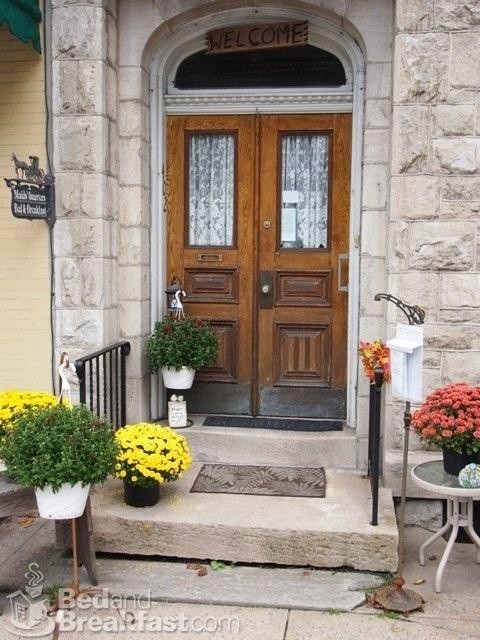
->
[202,416,343,432]
[189,463,326,498]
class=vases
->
[443,445,480,476]
[124,478,160,506]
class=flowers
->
[357,339,391,384]
[110,422,193,490]
[0,387,71,445]
[411,381,480,456]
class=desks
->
[409,460,480,593]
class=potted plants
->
[143,312,221,390]
[0,403,123,521]
